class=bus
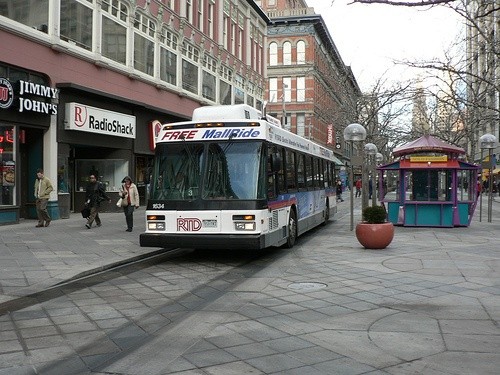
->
[139,104,346,251]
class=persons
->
[336,180,344,202]
[86,175,111,229]
[119,176,140,232]
[477,178,481,198]
[368,178,373,199]
[34,169,53,227]
[355,178,362,196]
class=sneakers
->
[44,220,51,227]
[94,223,102,227]
[85,224,91,229]
[35,223,44,227]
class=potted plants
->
[356,206,394,248]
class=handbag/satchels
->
[116,185,125,207]
[81,203,91,218]
